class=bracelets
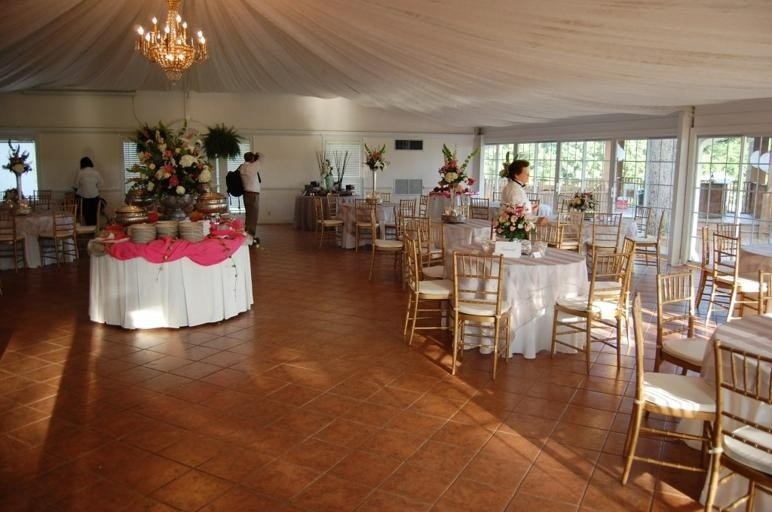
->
[366,198,383,204]
[441,215,465,222]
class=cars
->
[481,237,548,257]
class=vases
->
[326,174,334,193]
[320,158,334,175]
[362,142,391,172]
[123,106,213,201]
[3,139,31,176]
[365,169,383,205]
[14,172,23,203]
[425,144,482,199]
[156,193,194,220]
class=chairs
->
[295,183,772,380]
[644,269,708,418]
[622,292,716,486]
[0,186,103,276]
[703,340,772,512]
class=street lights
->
[227,171,244,197]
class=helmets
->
[128,220,203,243]
[101,237,129,244]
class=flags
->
[365,169,383,205]
[326,174,334,193]
[14,172,23,203]
[156,193,194,220]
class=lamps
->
[0,186,103,276]
[133,0,211,84]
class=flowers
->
[3,139,31,176]
[123,106,213,201]
[320,158,334,175]
[425,144,482,199]
[362,142,391,172]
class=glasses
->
[133,0,211,84]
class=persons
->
[239,152,264,245]
[73,157,103,226]
[502,160,548,226]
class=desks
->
[88,226,253,334]
[701,313,772,512]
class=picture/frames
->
[88,226,253,334]
